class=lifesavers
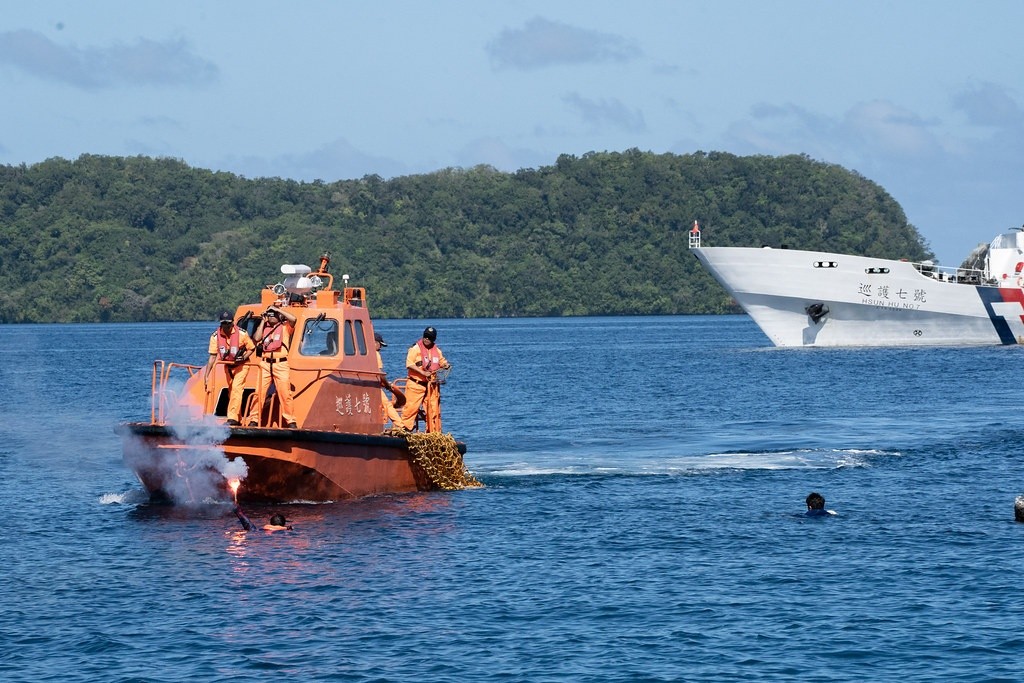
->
[1016,277,1024,289]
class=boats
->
[112,250,467,508]
[688,220,1024,347]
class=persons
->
[233,502,295,536]
[374,333,387,375]
[204,310,255,427]
[401,327,449,434]
[249,304,299,429]
[802,493,836,516]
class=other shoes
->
[227,419,242,426]
[287,422,297,429]
[248,421,258,427]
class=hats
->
[424,327,437,339]
[219,309,234,322]
[374,331,388,347]
[266,306,280,313]
